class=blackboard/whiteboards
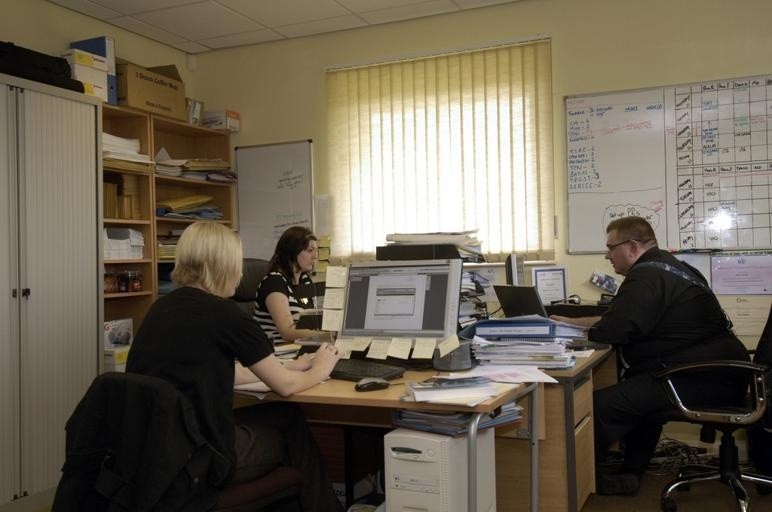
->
[561,71,772,258]
[233,139,315,259]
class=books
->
[385,231,466,245]
[472,335,577,368]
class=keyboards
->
[329,357,404,383]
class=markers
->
[686,245,716,255]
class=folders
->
[474,322,554,338]
[462,269,491,284]
[69,35,117,106]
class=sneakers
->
[595,472,641,497]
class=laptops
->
[492,284,549,318]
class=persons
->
[124,221,345,512]
[252,225,335,350]
[587,217,752,495]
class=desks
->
[226,362,546,512]
[470,339,615,512]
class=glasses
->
[606,238,641,251]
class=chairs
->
[60,366,318,512]
[639,296,772,511]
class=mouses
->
[355,377,389,392]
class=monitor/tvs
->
[505,253,525,286]
[339,257,463,341]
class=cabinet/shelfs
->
[1,74,103,511]
[97,94,238,354]
